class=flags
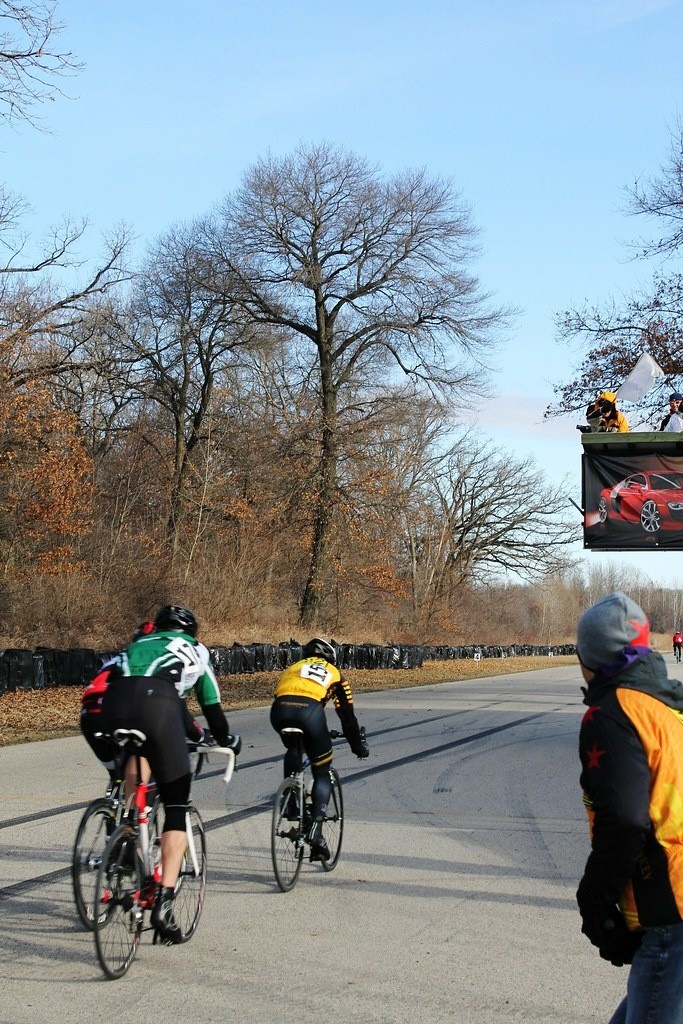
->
[616,351,663,404]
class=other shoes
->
[679,660,681,662]
[674,654,676,656]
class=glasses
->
[670,401,682,405]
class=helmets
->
[155,605,197,636]
[676,631,680,634]
[132,621,157,642]
[303,638,337,667]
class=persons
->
[659,391,683,432]
[576,591,683,1024]
[673,631,682,662]
[78,605,242,943]
[585,392,629,432]
[269,638,369,860]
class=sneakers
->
[304,821,330,861]
[283,786,300,821]
[150,897,183,944]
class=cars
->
[597,471,683,533]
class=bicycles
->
[91,728,236,981]
[673,647,683,663]
[269,726,367,893]
[70,733,137,931]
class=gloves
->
[581,910,643,965]
[361,741,369,758]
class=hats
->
[670,392,683,400]
[577,591,650,673]
